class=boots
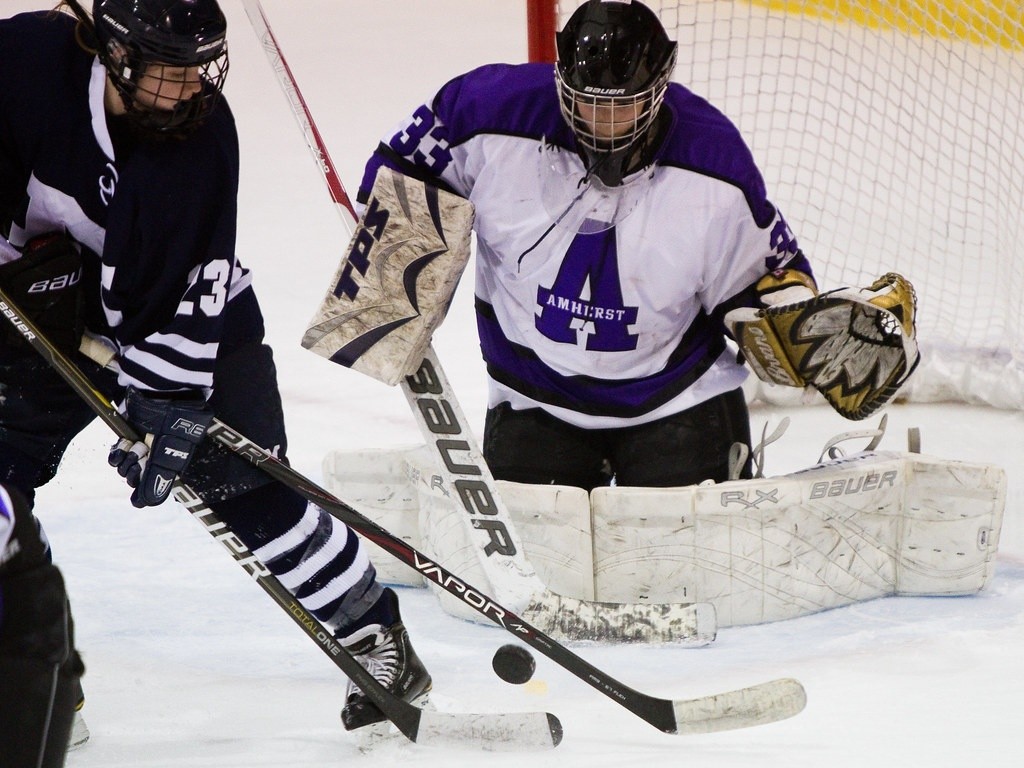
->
[333,589,434,762]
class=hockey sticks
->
[1,290,564,750]
[242,0,719,652]
[78,327,809,737]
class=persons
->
[0,3,430,768]
[358,16,827,486]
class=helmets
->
[92,0,230,137]
[553,0,678,153]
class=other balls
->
[491,645,539,686]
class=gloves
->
[106,388,212,511]
[1,239,85,376]
